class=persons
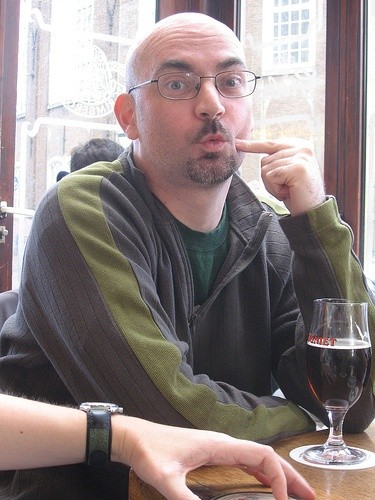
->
[70,138,126,173]
[0,11,375,499]
[0,391,316,500]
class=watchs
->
[79,402,124,470]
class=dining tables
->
[130,405,375,500]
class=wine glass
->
[303,298,373,466]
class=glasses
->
[127,69,261,101]
